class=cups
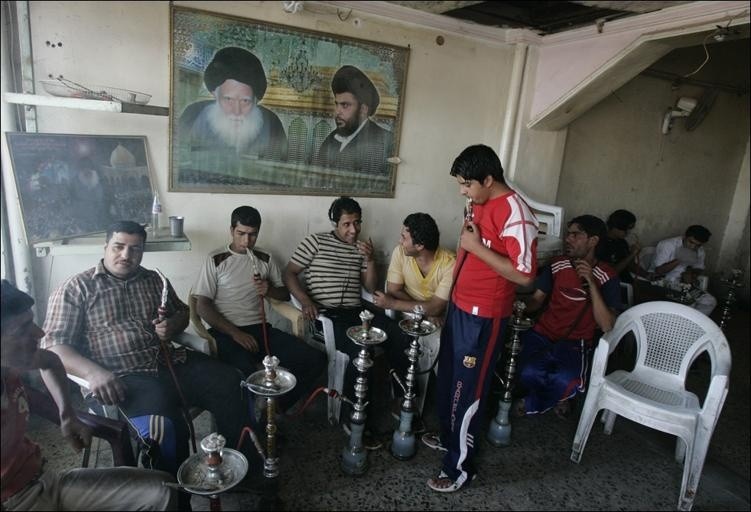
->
[169,216,185,237]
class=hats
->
[201,48,267,99]
[334,62,380,117]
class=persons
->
[419,145,542,495]
[35,219,255,503]
[314,61,395,177]
[177,44,291,163]
[649,221,719,321]
[195,203,329,427]
[513,211,626,427]
[280,196,428,449]
[601,201,645,306]
[2,278,190,511]
[369,213,486,453]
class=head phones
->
[330,199,344,227]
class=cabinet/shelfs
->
[5,90,190,252]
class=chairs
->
[569,247,735,301]
[460,180,565,301]
[2,247,569,512]
[571,300,733,511]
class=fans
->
[660,83,722,135]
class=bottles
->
[150,186,164,240]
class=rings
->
[375,300,377,303]
[90,392,100,399]
[305,310,310,316]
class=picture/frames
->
[166,3,412,199]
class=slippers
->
[425,467,478,495]
[421,430,449,454]
